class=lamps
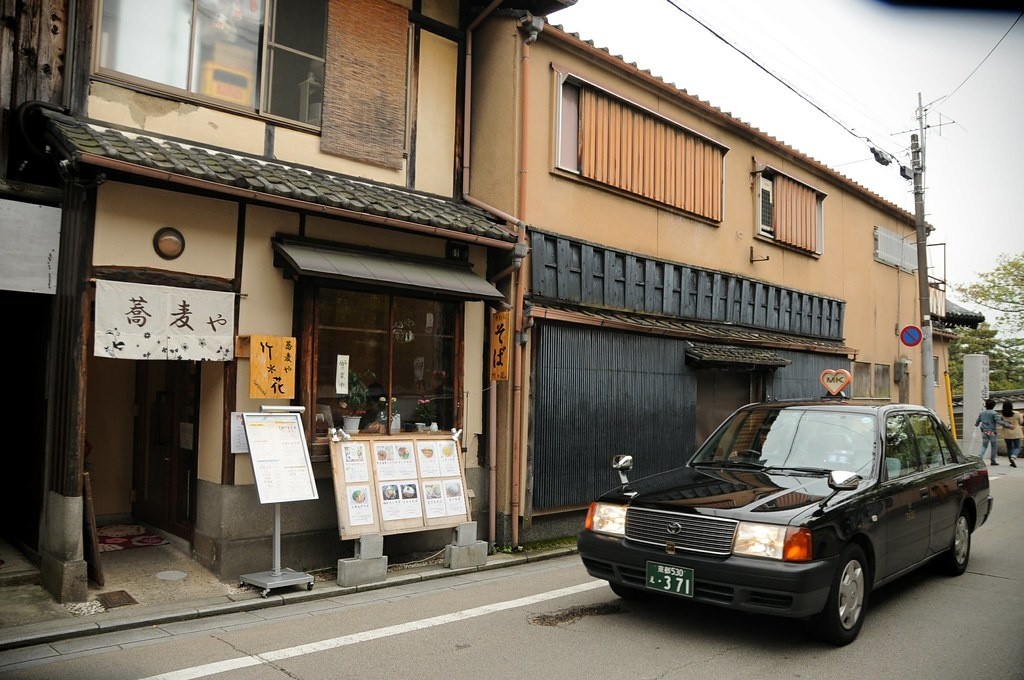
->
[152,225,186,260]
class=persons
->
[974,399,1014,465]
[1000,400,1024,468]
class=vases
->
[379,422,389,434]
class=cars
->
[578,396,991,647]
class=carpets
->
[96,521,171,553]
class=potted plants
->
[342,363,375,434]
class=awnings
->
[269,243,505,301]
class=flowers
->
[413,399,433,426]
[377,396,398,423]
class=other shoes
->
[991,462,999,465]
[1009,458,1017,467]
